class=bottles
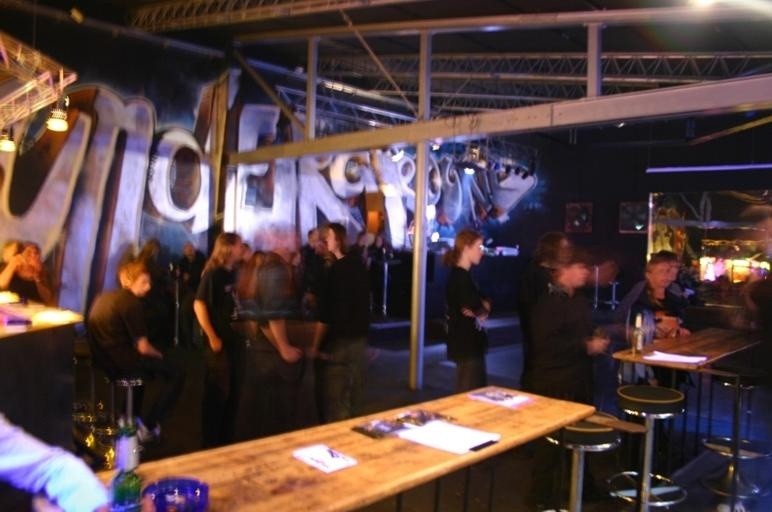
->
[111,420,142,512]
[632,312,644,356]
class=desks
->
[94,385,595,512]
[0,285,88,342]
[610,326,772,469]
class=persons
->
[83,222,394,463]
[2,412,113,511]
[519,229,710,512]
[0,238,57,307]
[441,226,493,394]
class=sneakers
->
[136,418,162,444]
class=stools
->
[115,376,145,433]
[543,409,622,512]
[607,383,688,512]
[700,370,772,512]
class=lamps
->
[45,91,71,132]
[1,126,21,153]
